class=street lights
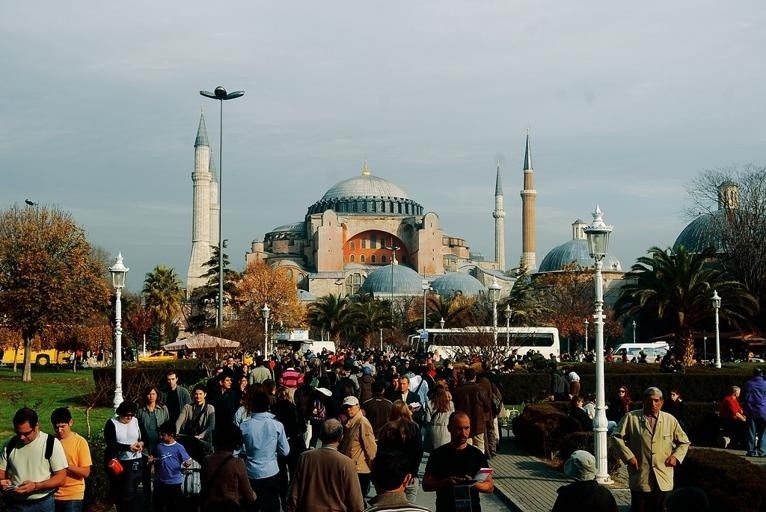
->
[503,303,511,350]
[439,317,444,341]
[200,85,244,339]
[23,195,38,220]
[710,288,725,368]
[632,317,637,341]
[419,280,427,330]
[489,275,499,350]
[631,320,636,343]
[584,204,614,485]
[385,245,399,330]
[259,301,282,363]
[202,295,230,363]
[582,318,588,352]
[107,250,128,408]
[703,329,709,363]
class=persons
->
[1,343,766,512]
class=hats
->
[343,394,358,407]
[563,449,597,483]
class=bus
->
[0,324,90,369]
[407,326,562,367]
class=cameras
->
[132,461,139,471]
[7,483,24,490]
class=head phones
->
[447,408,472,432]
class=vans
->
[606,341,669,364]
[301,339,337,359]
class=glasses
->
[13,429,33,436]
[126,413,134,416]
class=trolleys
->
[145,433,212,510]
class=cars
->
[733,356,765,364]
[138,349,186,365]
